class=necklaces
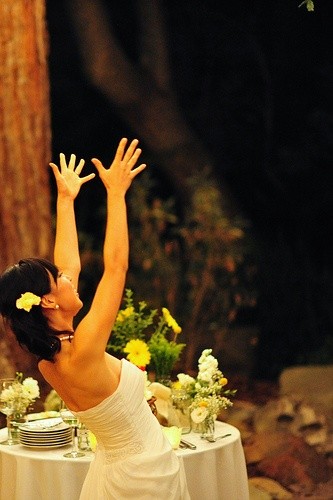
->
[59,336,73,341]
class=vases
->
[189,407,215,445]
[8,412,27,445]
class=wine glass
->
[59,410,86,457]
[0,378,19,446]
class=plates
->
[19,418,73,449]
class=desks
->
[0,420,250,500]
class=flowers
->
[105,290,186,389]
[177,349,237,438]
[0,377,41,439]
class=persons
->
[0,138,189,500]
